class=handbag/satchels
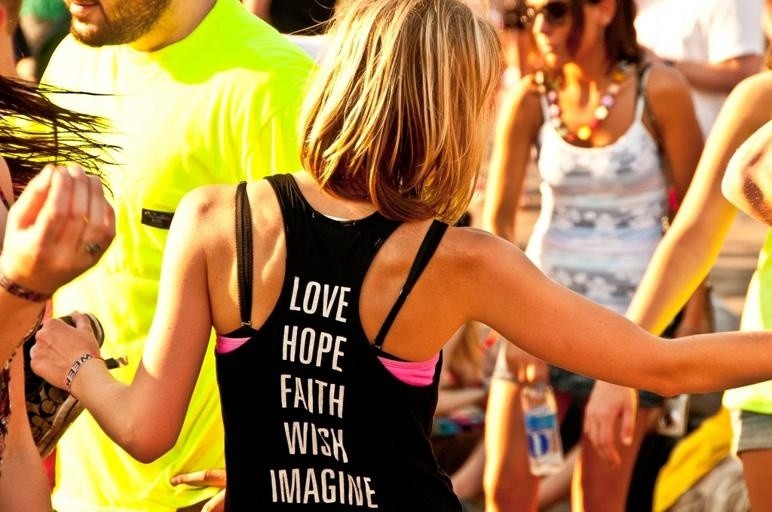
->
[1,310,105,474]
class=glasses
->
[515,1,567,22]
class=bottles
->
[520,376,568,474]
